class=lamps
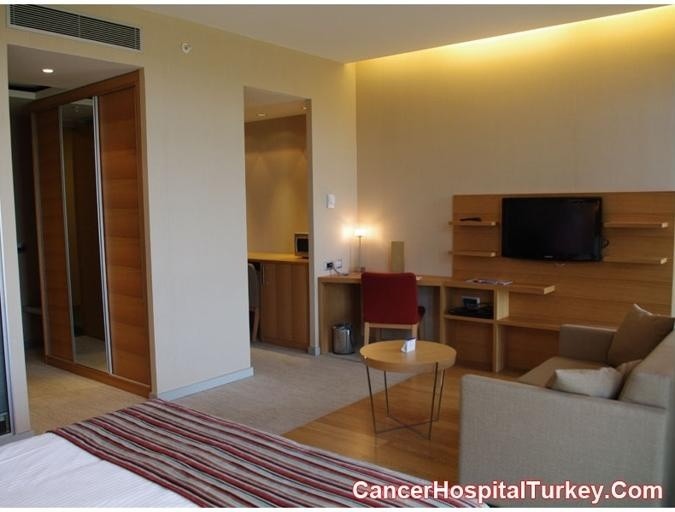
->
[352,221,376,274]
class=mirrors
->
[58,97,113,379]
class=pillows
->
[608,303,671,370]
[549,359,644,402]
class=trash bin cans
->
[332,326,355,354]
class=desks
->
[361,336,456,439]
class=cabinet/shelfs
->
[447,220,667,333]
[264,260,311,355]
[318,276,504,372]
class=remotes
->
[460,217,481,221]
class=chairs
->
[248,264,262,341]
[362,271,425,344]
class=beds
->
[1,399,495,506]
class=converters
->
[327,263,333,267]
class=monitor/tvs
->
[501,196,602,262]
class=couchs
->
[459,302,673,506]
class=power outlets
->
[325,261,334,270]
